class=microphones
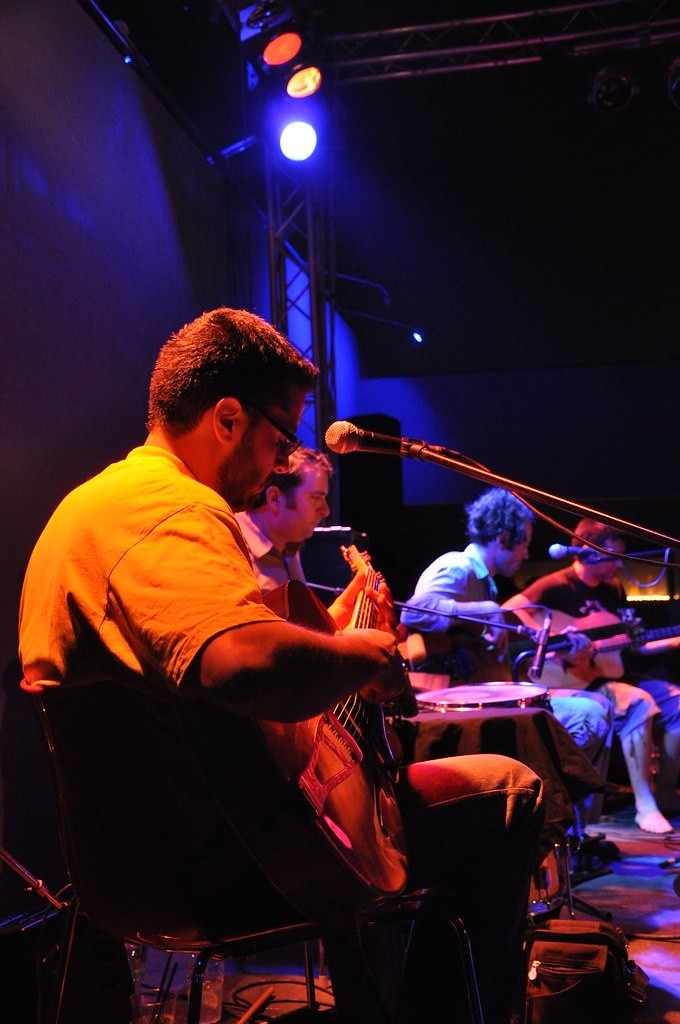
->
[324,421,452,455]
[527,618,552,682]
[549,543,615,560]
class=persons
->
[17,306,543,1024]
[233,449,393,653]
[499,518,680,833]
[400,490,612,843]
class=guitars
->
[168,543,418,924]
[395,604,680,690]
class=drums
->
[415,679,565,919]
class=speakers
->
[293,526,367,607]
[0,905,137,1024]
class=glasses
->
[251,400,303,456]
